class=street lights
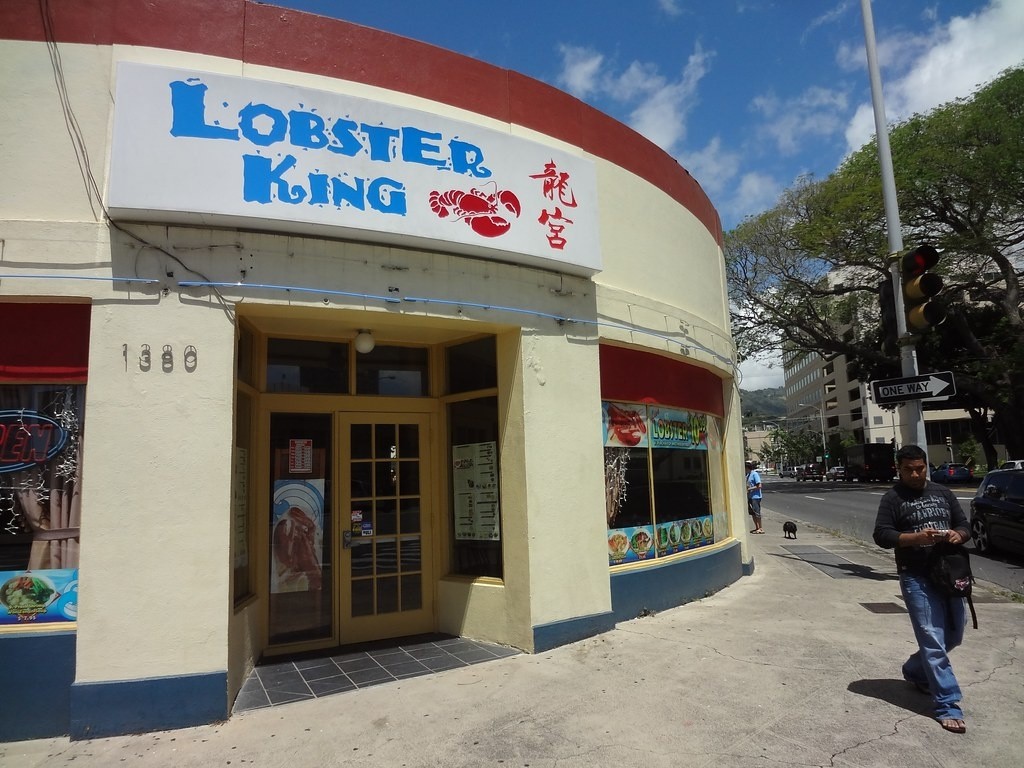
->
[762,420,783,473]
[798,403,828,471]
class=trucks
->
[843,442,896,483]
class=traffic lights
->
[824,449,829,458]
[946,437,952,447]
[902,244,946,333]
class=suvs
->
[970,469,1024,561]
[779,466,797,478]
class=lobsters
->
[273,507,322,607]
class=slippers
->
[942,718,966,733]
[902,666,932,695]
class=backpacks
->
[930,541,979,630]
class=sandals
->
[750,528,765,533]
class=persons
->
[871,444,975,732]
[744,462,769,532]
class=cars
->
[825,466,845,481]
[796,467,823,482]
[754,466,774,473]
[995,460,1024,469]
[931,462,974,484]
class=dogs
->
[783,521,798,539]
